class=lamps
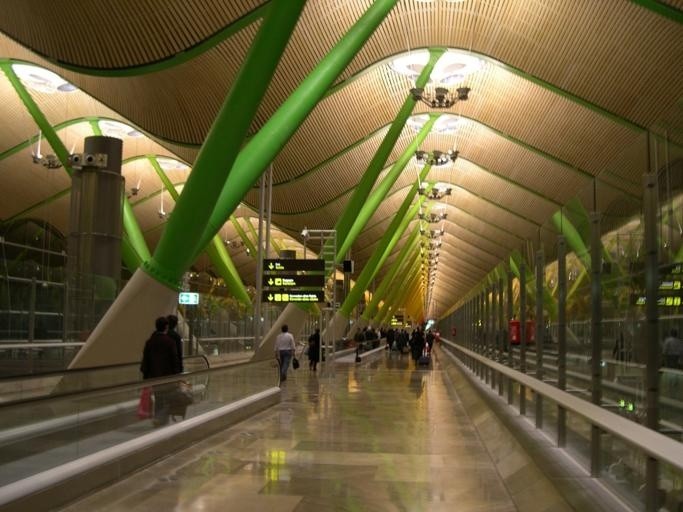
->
[406,81,472,288]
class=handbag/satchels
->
[293,358,299,369]
[178,381,193,399]
[137,387,153,418]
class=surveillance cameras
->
[85,153,96,164]
[72,153,84,165]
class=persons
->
[273,324,295,382]
[353,325,435,367]
[166,315,184,374]
[139,315,180,430]
[307,328,324,371]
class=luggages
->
[391,345,398,350]
[170,391,188,420]
[403,347,409,353]
[419,350,429,366]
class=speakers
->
[343,260,354,273]
